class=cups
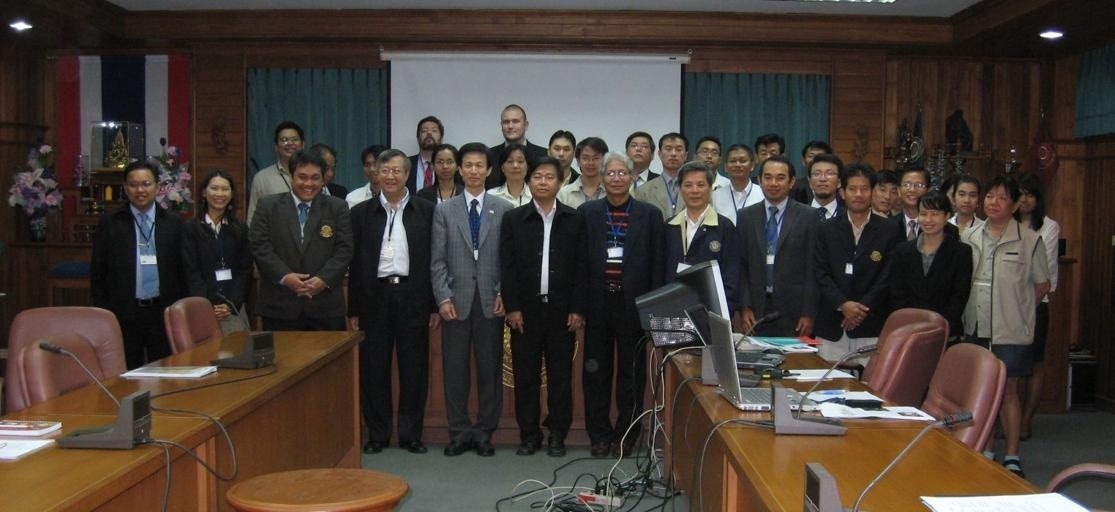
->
[436,159,456,167]
[377,167,407,176]
[279,136,303,145]
[604,170,629,177]
[809,169,839,178]
[628,143,650,152]
[899,183,927,190]
[125,179,156,189]
[697,149,720,157]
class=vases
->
[7,145,64,219]
[141,138,194,212]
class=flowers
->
[38,341,121,414]
[736,312,782,350]
[796,344,886,418]
[215,292,251,335]
[850,411,974,512]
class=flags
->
[30,217,47,242]
[172,201,190,213]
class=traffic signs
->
[608,485,629,507]
[579,492,611,507]
[627,482,646,491]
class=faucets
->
[1002,460,1025,479]
[992,456,998,462]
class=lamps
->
[921,342,1006,454]
[864,308,948,411]
[164,296,218,353]
[1046,463,1115,509]
[5,306,124,413]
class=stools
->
[376,276,409,285]
[541,294,550,306]
[603,283,624,296]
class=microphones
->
[226,469,409,512]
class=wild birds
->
[680,304,786,369]
[708,308,814,413]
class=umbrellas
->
[591,441,611,457]
[518,440,543,456]
[548,437,566,457]
[1020,432,1030,440]
[994,431,1004,440]
[398,438,427,453]
[611,436,631,457]
[363,438,390,454]
[444,438,473,456]
[471,437,495,457]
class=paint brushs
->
[580,487,620,506]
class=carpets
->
[765,206,778,288]
[424,161,433,189]
[907,220,916,242]
[817,207,828,224]
[137,212,159,294]
[469,199,483,250]
[297,202,309,225]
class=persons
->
[249,149,351,333]
[816,162,896,340]
[751,135,785,170]
[692,138,731,190]
[578,152,664,456]
[959,180,1051,480]
[500,157,583,458]
[869,168,898,216]
[557,138,609,210]
[346,144,386,209]
[313,143,348,199]
[896,165,959,241]
[248,123,305,226]
[660,161,738,326]
[806,151,845,220]
[631,134,688,219]
[549,129,580,191]
[417,145,463,204]
[1015,174,1060,440]
[90,161,185,370]
[488,148,535,210]
[792,141,833,205]
[182,170,254,340]
[889,192,972,346]
[407,115,445,197]
[489,106,550,188]
[950,178,982,229]
[941,178,960,213]
[431,143,514,457]
[350,149,441,454]
[710,145,765,224]
[626,131,660,194]
[737,155,816,338]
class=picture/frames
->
[650,338,1043,512]
[0,329,363,512]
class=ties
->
[632,262,732,359]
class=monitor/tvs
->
[921,491,1089,511]
[121,366,218,378]
[1,420,62,436]
